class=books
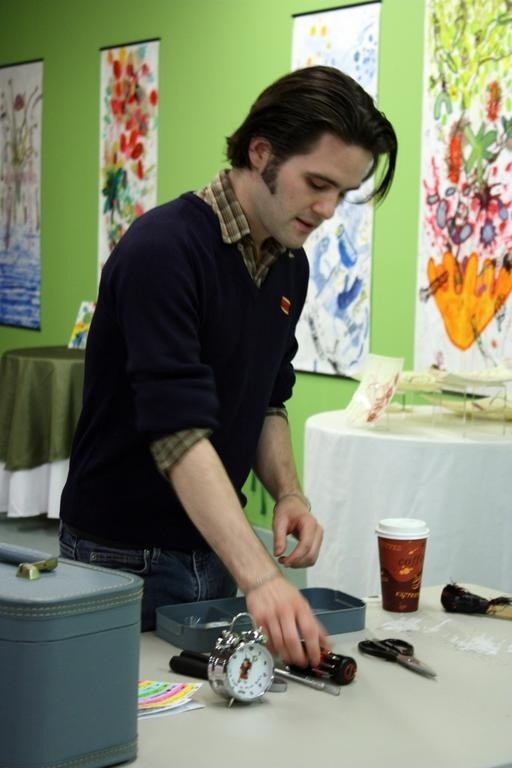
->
[137,679,206,722]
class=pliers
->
[169,649,288,692]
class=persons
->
[59,66,398,668]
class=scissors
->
[359,639,437,678]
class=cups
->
[374,517,432,612]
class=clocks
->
[205,610,276,710]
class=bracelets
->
[273,493,311,512]
[244,571,286,597]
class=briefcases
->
[0,543,142,768]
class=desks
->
[129,576,510,768]
[302,407,512,576]
[0,345,84,521]
